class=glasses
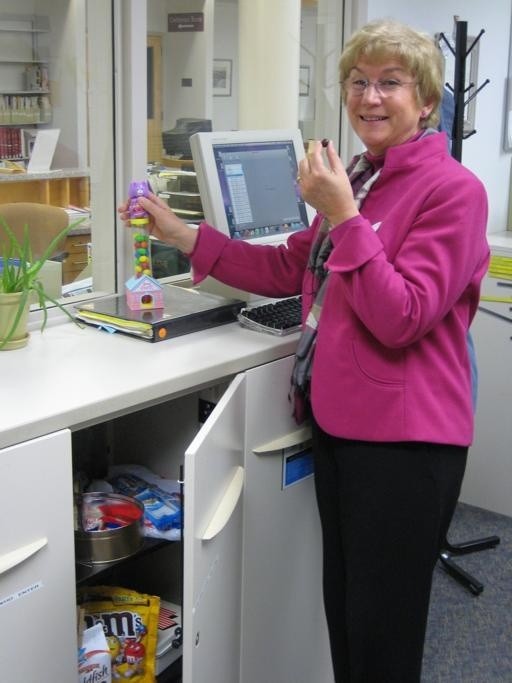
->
[339,75,420,98]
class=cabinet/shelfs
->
[1,372,249,683]
[63,234,92,284]
[249,352,332,683]
[0,14,50,130]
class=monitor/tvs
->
[161,117,212,160]
[187,128,318,248]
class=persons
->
[115,16,490,680]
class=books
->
[0,62,54,162]
[486,244,511,281]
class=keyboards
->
[236,296,303,337]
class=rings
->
[296,176,303,184]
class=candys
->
[323,138,329,147]
[133,233,151,279]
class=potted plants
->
[1,213,87,351]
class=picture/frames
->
[299,65,310,96]
[212,58,234,96]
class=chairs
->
[1,201,70,288]
